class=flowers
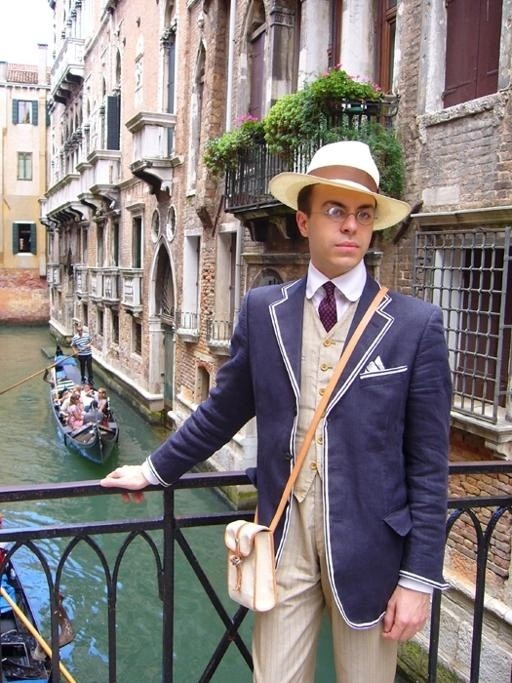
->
[262,64,384,162]
[201,113,261,183]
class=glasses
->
[307,205,379,226]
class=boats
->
[1,523,74,683]
[43,334,121,465]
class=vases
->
[325,94,362,112]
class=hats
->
[267,139,411,231]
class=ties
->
[319,281,337,332]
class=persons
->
[97,140,455,682]
[70,325,94,384]
[52,383,110,431]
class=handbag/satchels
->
[224,517,278,611]
